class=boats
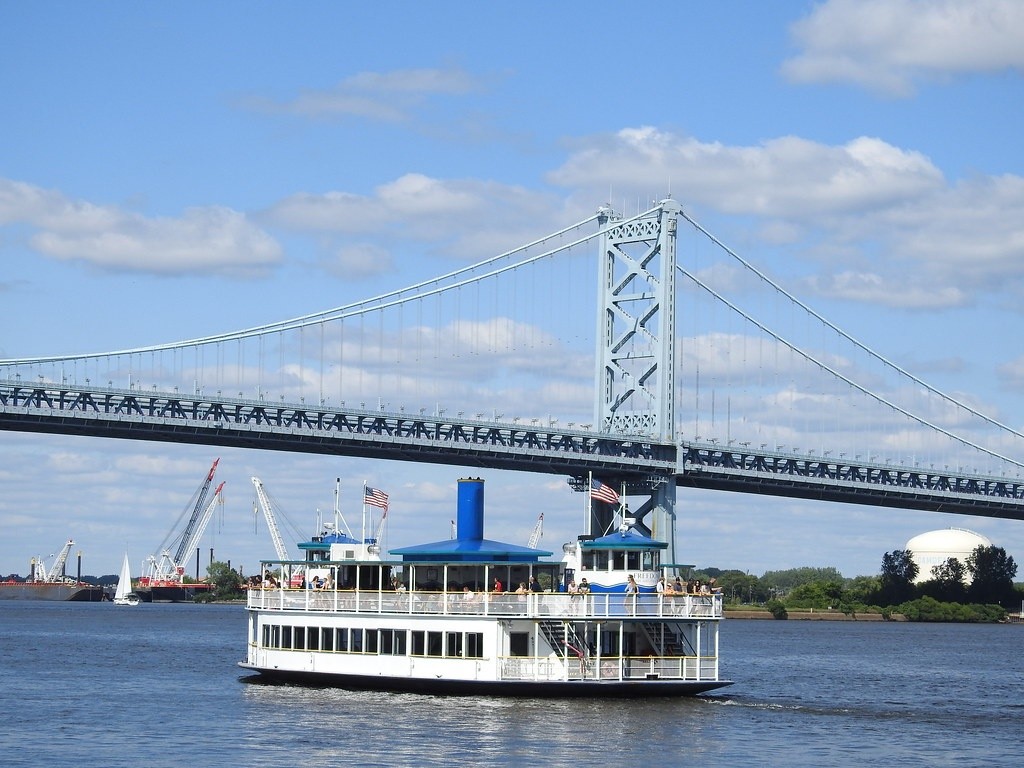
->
[232,470,736,695]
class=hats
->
[667,583,671,587]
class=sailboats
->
[113,548,140,606]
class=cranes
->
[36,540,76,583]
[147,454,308,583]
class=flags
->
[590,477,619,505]
[365,486,389,510]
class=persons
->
[250,569,723,617]
